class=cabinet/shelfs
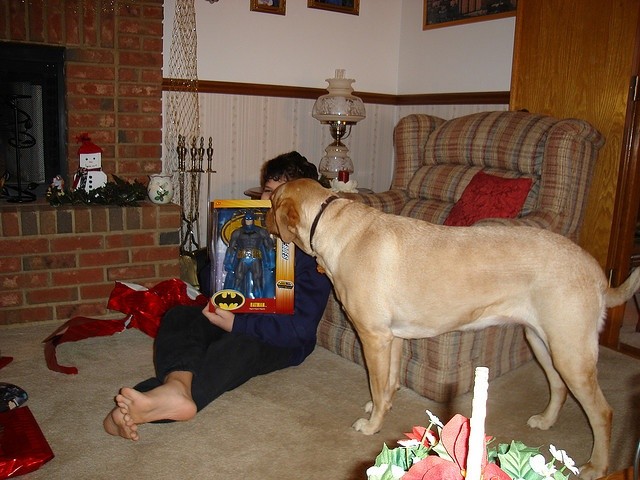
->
[510,1,640,356]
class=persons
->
[103,151,332,441]
[222,210,274,299]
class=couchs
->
[314,102,607,405]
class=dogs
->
[265,176,640,480]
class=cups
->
[146,172,175,204]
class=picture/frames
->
[307,1,361,17]
[247,0,287,16]
[422,0,518,32]
